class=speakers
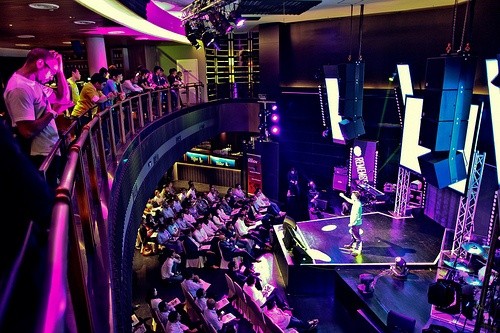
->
[339,62,366,140]
[387,311,416,333]
[283,215,296,229]
[418,54,476,189]
[292,246,313,264]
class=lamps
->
[185,9,246,51]
[358,274,375,295]
[281,214,313,263]
[324,52,499,190]
[391,256,407,272]
[426,280,457,308]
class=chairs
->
[131,180,301,333]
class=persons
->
[339,190,362,255]
[132,181,319,333]
[288,168,300,205]
[3,47,70,185]
[60,63,183,153]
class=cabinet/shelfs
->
[51,49,87,79]
[108,48,129,74]
